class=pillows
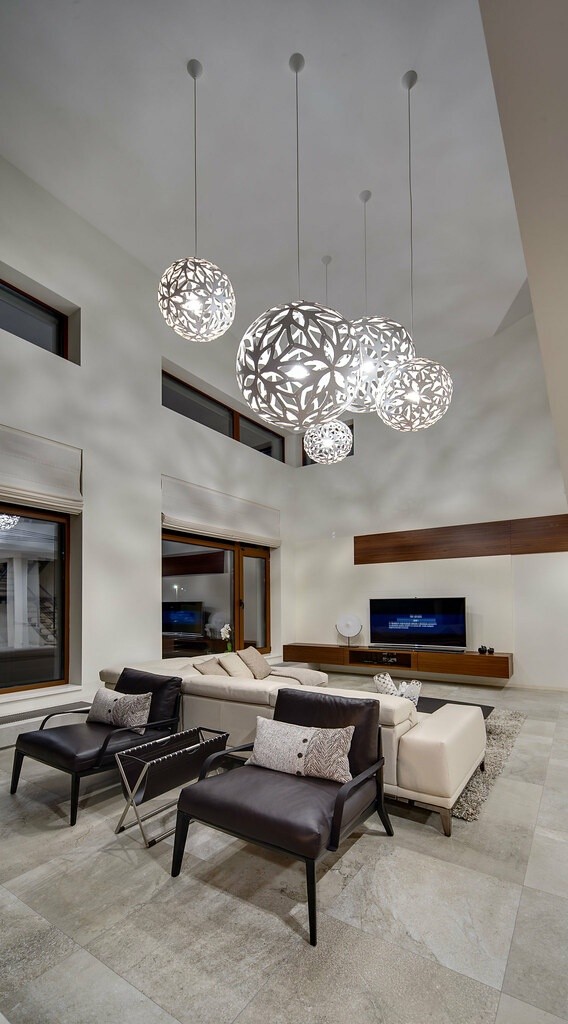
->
[218,651,255,679]
[178,664,202,675]
[86,686,153,736]
[236,645,273,680]
[244,716,356,784]
[192,657,230,676]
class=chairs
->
[10,668,183,826]
[172,689,395,947]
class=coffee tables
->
[374,693,495,772]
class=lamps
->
[157,57,236,342]
[345,190,416,414]
[374,72,453,433]
[0,512,21,533]
[303,254,353,464]
[236,55,363,434]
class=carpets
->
[383,693,527,824]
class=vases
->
[226,642,232,652]
[205,648,217,655]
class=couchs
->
[99,666,488,837]
[128,652,329,686]
[0,644,61,687]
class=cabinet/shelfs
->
[283,643,514,678]
[162,636,256,658]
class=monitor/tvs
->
[369,598,466,652]
[162,602,202,635]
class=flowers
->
[220,624,231,642]
[205,624,219,648]
